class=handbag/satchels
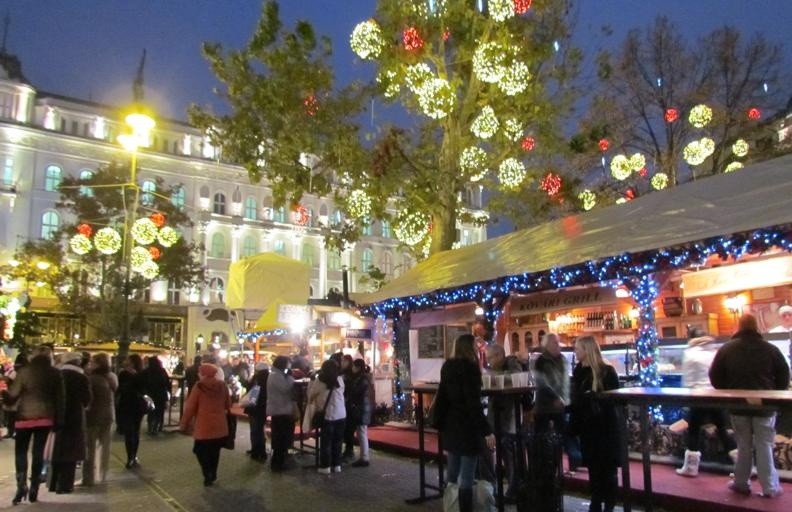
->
[220,408,236,450]
[303,391,316,432]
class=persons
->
[767,305,792,368]
[535,332,583,473]
[117,354,149,470]
[350,359,375,467]
[74,353,118,488]
[46,351,91,494]
[186,356,201,389]
[180,363,233,487]
[485,344,522,503]
[435,334,496,511]
[309,359,346,474]
[292,350,311,377]
[676,326,758,481]
[568,336,630,512]
[144,353,171,437]
[341,355,358,458]
[1,345,64,503]
[174,362,186,381]
[266,356,296,471]
[708,314,790,498]
[330,352,343,372]
[239,362,269,461]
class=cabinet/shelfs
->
[656,312,719,337]
[546,306,638,336]
[529,332,792,480]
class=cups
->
[481,375,491,389]
[495,376,505,389]
[521,373,529,388]
[511,373,520,388]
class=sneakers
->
[204,477,215,486]
[343,451,354,457]
[12,435,16,439]
[764,487,784,497]
[74,479,94,486]
[134,456,138,464]
[2,433,13,439]
[76,461,81,469]
[126,456,135,468]
[116,426,126,435]
[318,467,331,474]
[56,488,74,493]
[48,487,56,491]
[727,480,752,494]
[330,465,341,472]
[353,461,369,467]
[252,452,267,459]
[270,453,295,471]
[246,450,253,454]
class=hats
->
[61,351,83,363]
[255,362,268,371]
[200,363,217,377]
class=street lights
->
[9,232,50,314]
[115,108,156,378]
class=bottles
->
[557,314,585,332]
[602,309,618,329]
[624,316,628,328]
[585,312,603,328]
[627,314,631,327]
[619,314,625,329]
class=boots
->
[13,472,28,504]
[153,422,160,436]
[675,449,701,477]
[29,475,40,502]
[146,423,155,435]
[459,488,473,512]
[729,448,757,478]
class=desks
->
[403,383,538,512]
[603,387,792,512]
